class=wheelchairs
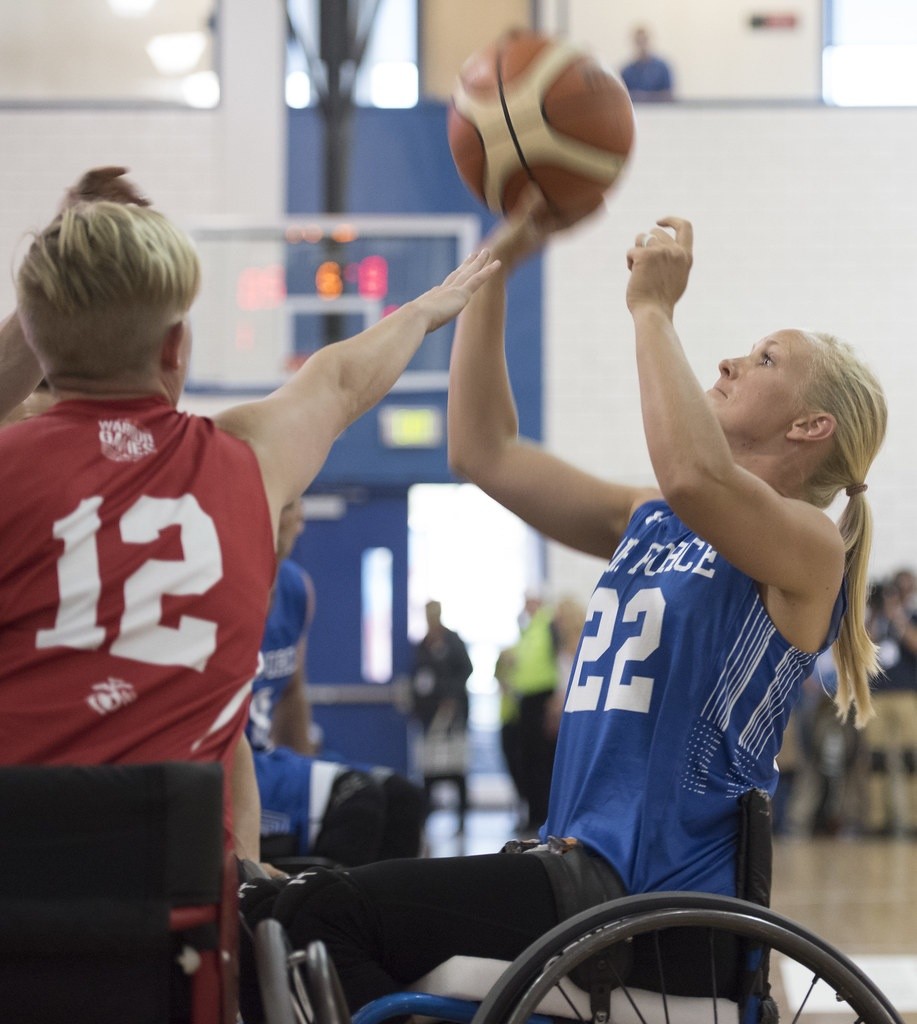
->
[249,788,908,1024]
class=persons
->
[619,25,672,99]
[0,199,504,1024]
[0,148,318,879]
[494,578,587,838]
[399,600,473,838]
[280,189,886,1024]
[769,567,917,841]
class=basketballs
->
[450,25,636,232]
